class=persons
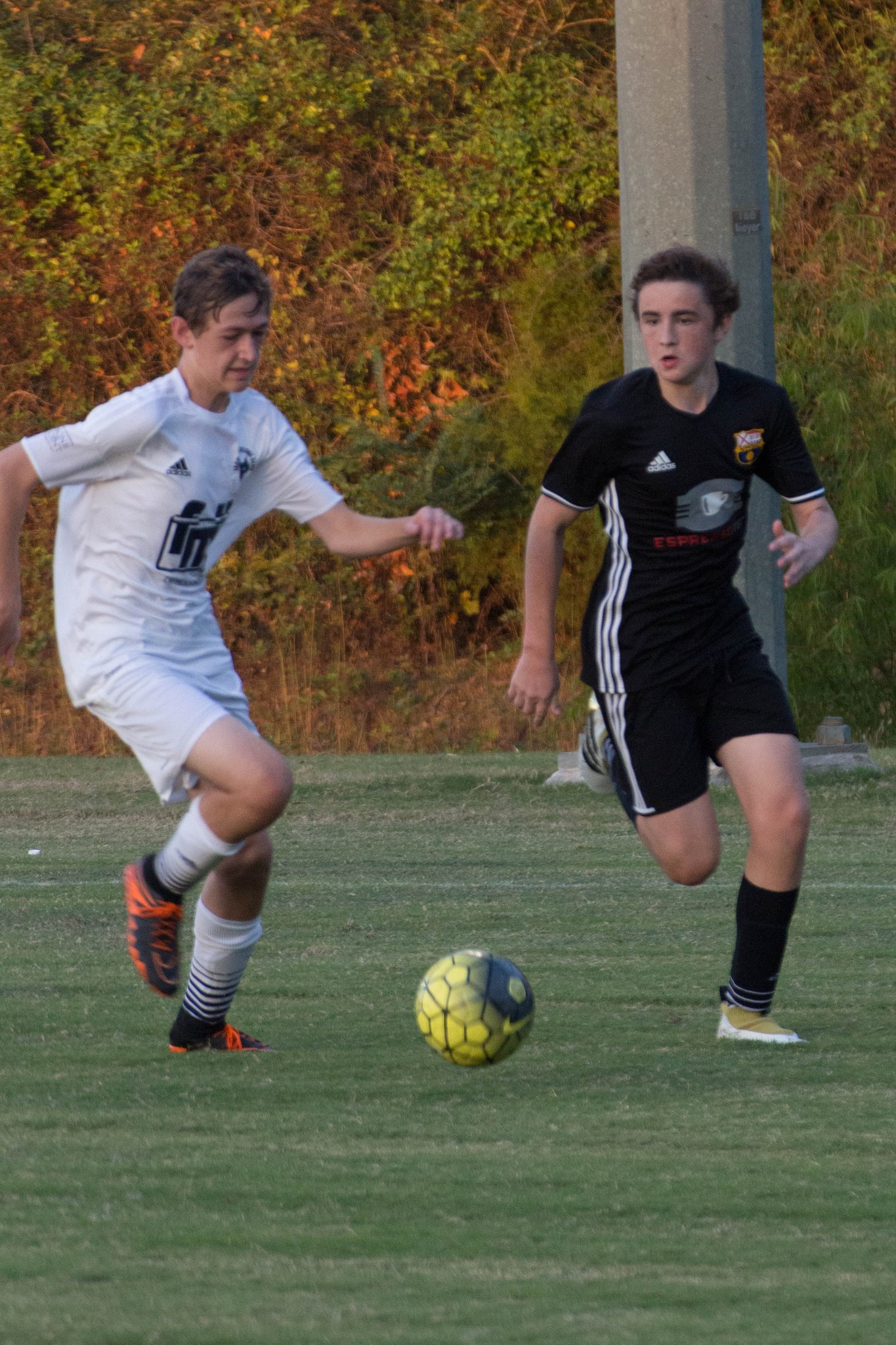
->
[506,245,835,1045]
[0,245,463,1054]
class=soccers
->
[416,948,540,1063]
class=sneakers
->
[169,1008,273,1053]
[716,1001,799,1045]
[124,854,181,997]
[578,690,616,795]
